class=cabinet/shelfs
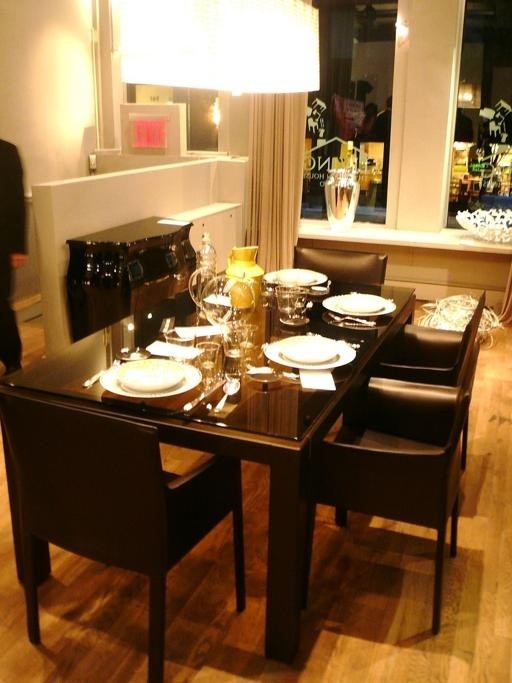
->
[65,215,198,342]
[171,202,240,285]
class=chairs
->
[0,382,247,683]
[291,245,489,635]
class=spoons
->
[215,379,242,411]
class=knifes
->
[183,378,226,411]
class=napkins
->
[175,325,229,342]
[299,368,336,391]
[146,339,206,360]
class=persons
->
[362,103,379,135]
[369,96,392,208]
[454,108,473,142]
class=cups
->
[275,285,309,326]
[164,312,257,387]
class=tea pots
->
[224,245,267,310]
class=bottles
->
[194,233,220,310]
[325,167,361,227]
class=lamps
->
[115,0,323,95]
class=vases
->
[322,168,361,234]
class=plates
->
[264,360,354,384]
[94,359,202,399]
[322,293,397,317]
[267,335,356,370]
[324,308,395,332]
[263,268,330,286]
